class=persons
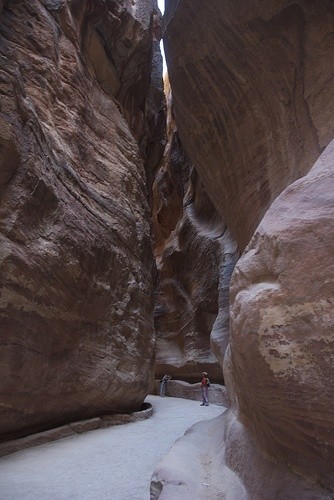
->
[200,372,209,406]
[160,374,172,397]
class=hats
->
[201,372,208,375]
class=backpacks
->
[205,378,210,387]
